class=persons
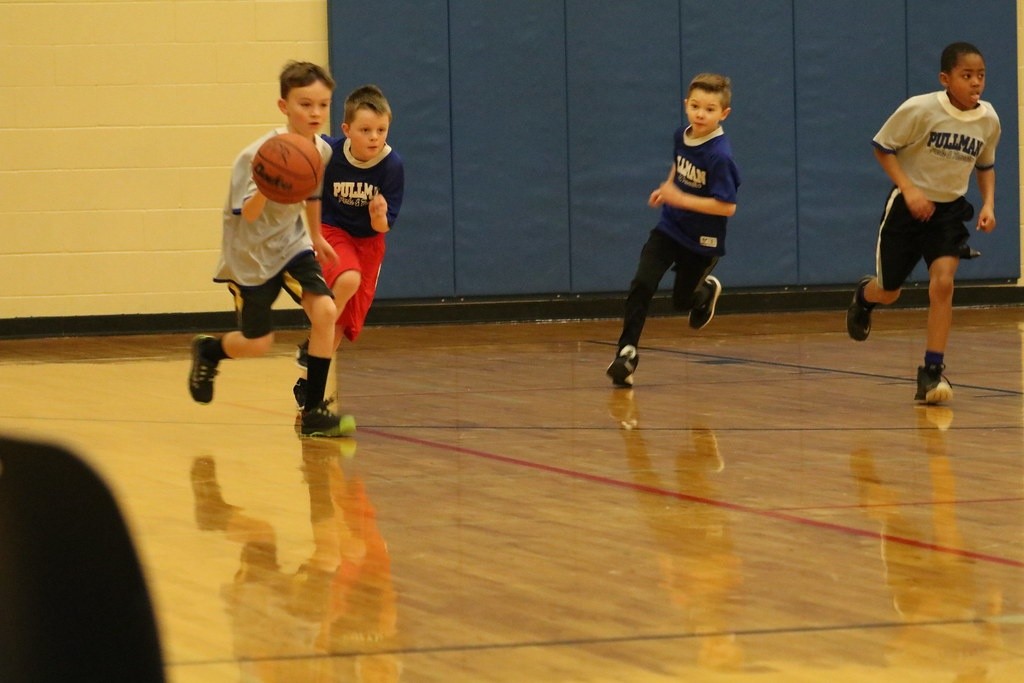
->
[856,408,1005,682]
[189,441,404,683]
[604,388,747,676]
[605,71,740,388]
[188,59,406,437]
[846,40,1002,408]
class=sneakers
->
[607,343,638,388]
[687,275,722,331]
[846,275,875,342]
[292,377,309,410]
[295,336,309,371]
[914,362,954,404]
[187,334,220,405]
[301,402,357,438]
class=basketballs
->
[252,133,326,204]
